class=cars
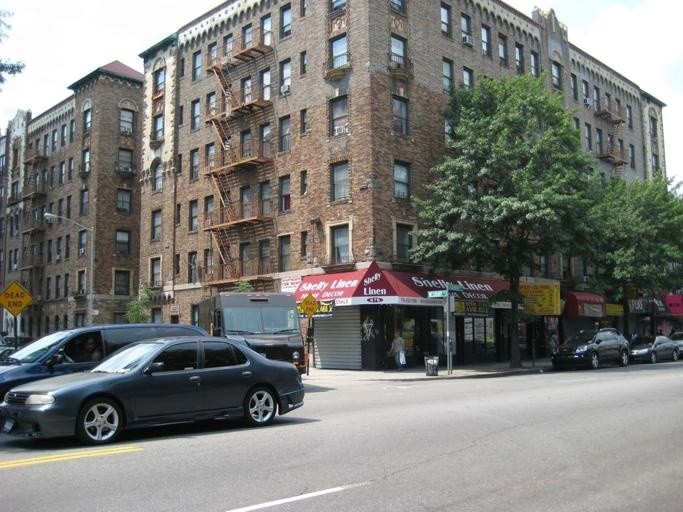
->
[629,331,682,364]
[0,336,305,444]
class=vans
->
[552,327,629,369]
[0,323,207,404]
[197,291,306,376]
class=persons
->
[390,331,407,371]
[78,337,102,361]
[631,330,638,339]
[548,330,560,359]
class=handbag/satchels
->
[400,351,406,364]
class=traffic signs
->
[428,290,447,298]
[301,292,318,320]
[0,280,31,315]
[448,283,463,291]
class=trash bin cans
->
[424,355,440,377]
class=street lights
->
[44,212,94,325]
